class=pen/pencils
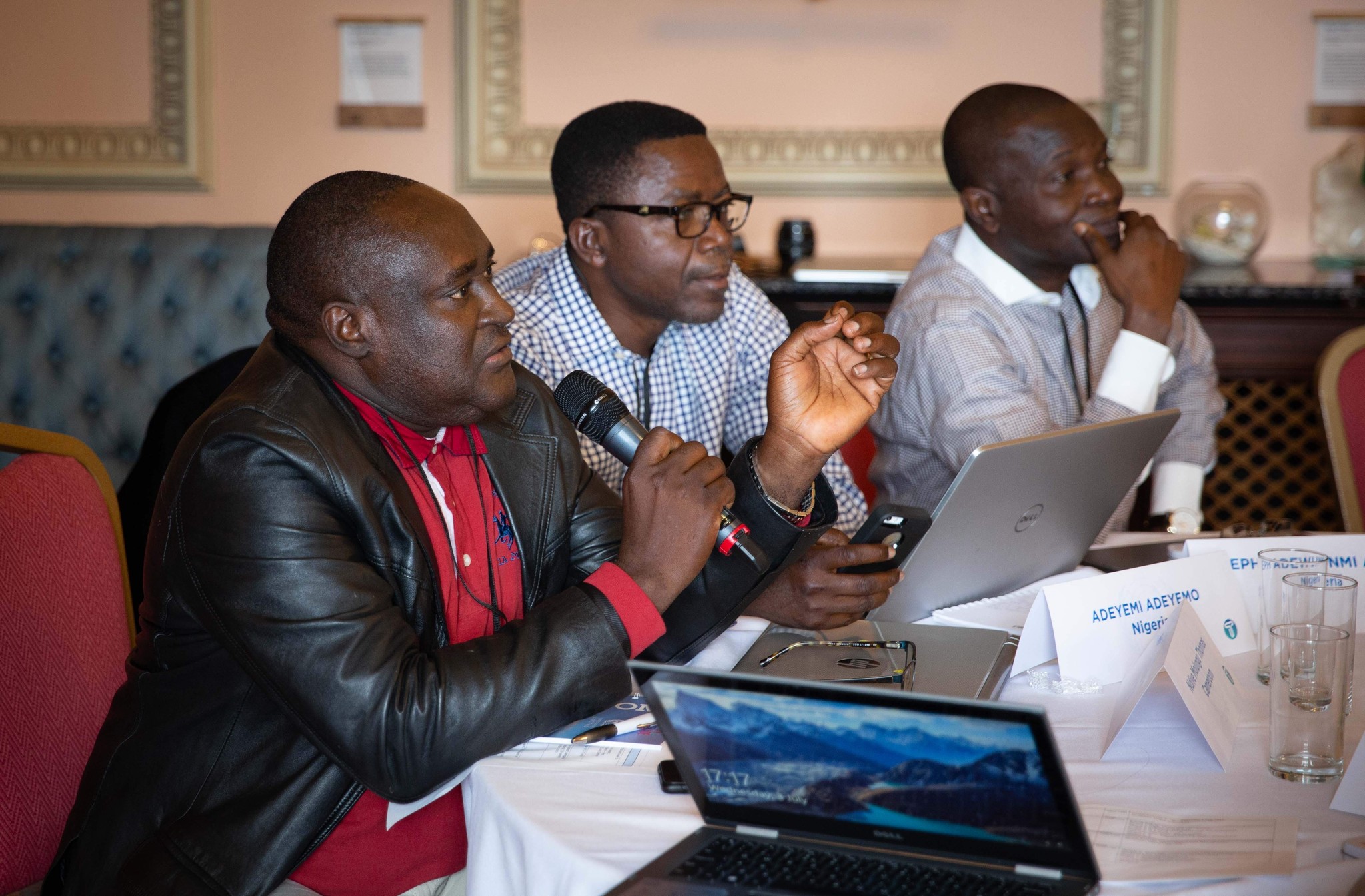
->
[570,713,658,744]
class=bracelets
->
[749,438,815,522]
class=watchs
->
[1150,510,1204,536]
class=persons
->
[866,81,1228,554]
[34,170,899,896]
[494,100,907,633]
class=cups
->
[1257,548,1329,685]
[779,219,814,275]
[1283,572,1358,716]
[1270,623,1350,785]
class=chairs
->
[0,310,1365,896]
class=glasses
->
[583,192,754,240]
[759,639,917,696]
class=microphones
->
[554,370,773,575]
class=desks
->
[462,533,1365,896]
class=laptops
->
[599,660,1103,896]
[731,617,1020,704]
[867,409,1181,623]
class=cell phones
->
[837,502,932,575]
[656,760,690,794]
